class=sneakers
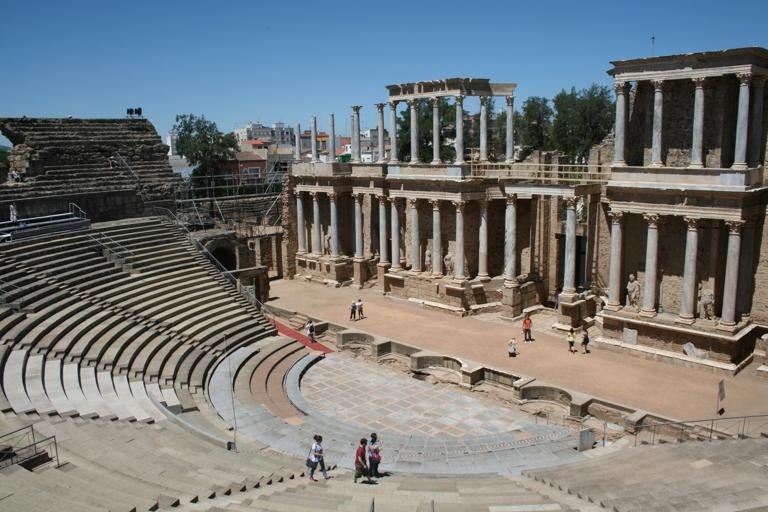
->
[325,475,332,480]
[309,475,314,480]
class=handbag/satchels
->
[371,456,381,464]
[306,457,318,469]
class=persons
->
[521,314,532,343]
[304,319,314,339]
[12,169,22,182]
[568,326,589,354]
[350,299,364,320]
[308,435,332,481]
[507,336,520,355]
[9,202,17,223]
[354,433,383,483]
